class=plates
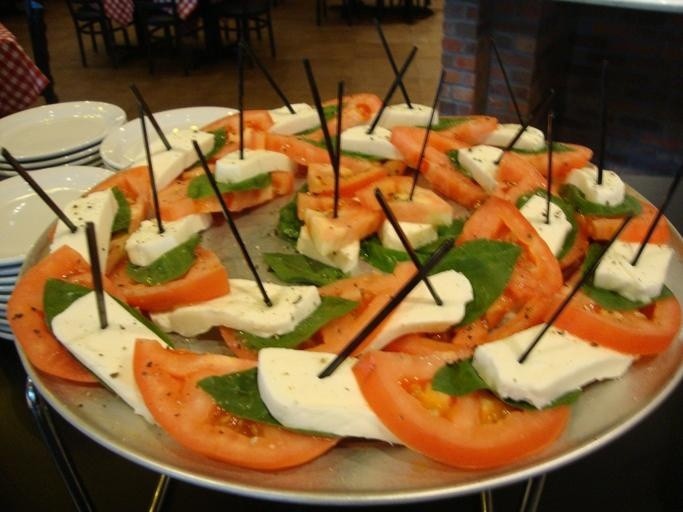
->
[0,100,683,506]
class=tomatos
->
[7,93,679,471]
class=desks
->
[0,23,51,118]
